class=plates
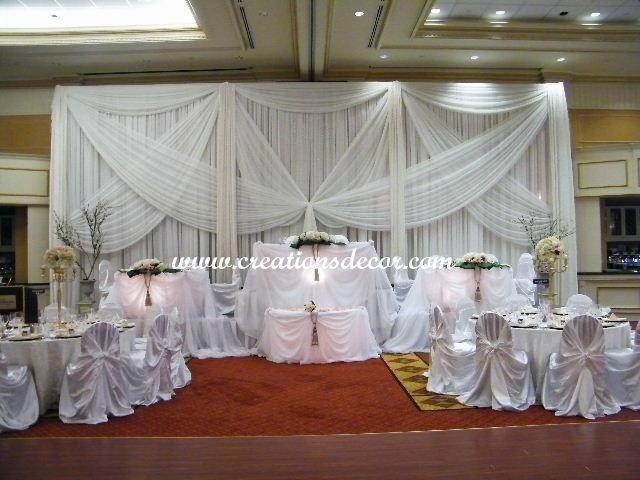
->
[472,310,625,331]
[6,322,135,342]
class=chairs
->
[427,294,640,420]
[98,235,534,366]
[0,304,195,431]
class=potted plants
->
[52,198,113,295]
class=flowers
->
[286,230,350,246]
[534,236,568,273]
[42,245,78,281]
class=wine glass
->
[9,311,24,330]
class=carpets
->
[0,354,640,438]
